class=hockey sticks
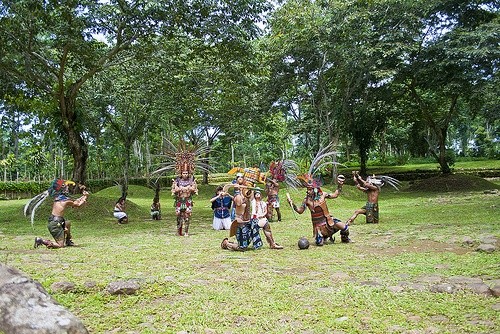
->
[223,184,264,192]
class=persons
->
[34,179,89,250]
[114,197,128,225]
[151,198,161,221]
[210,166,380,253]
[171,169,197,237]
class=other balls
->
[298,238,309,249]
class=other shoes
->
[221,238,228,249]
[271,243,283,249]
[342,238,356,243]
[34,237,43,249]
